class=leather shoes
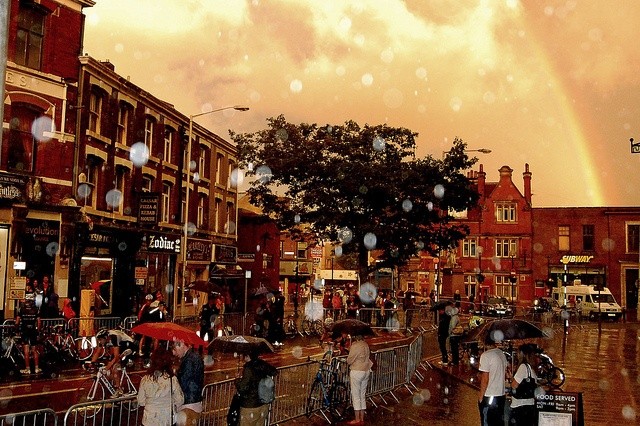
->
[360,421,364,426]
[347,420,360,426]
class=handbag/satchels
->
[451,316,464,334]
[510,363,537,399]
[226,390,241,426]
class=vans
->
[566,285,623,321]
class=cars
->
[485,296,515,318]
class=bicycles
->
[306,356,348,421]
[318,341,349,378]
[0,323,138,373]
[303,315,329,336]
[503,348,565,387]
[76,361,140,419]
[284,310,304,338]
[448,341,487,360]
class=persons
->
[264,293,287,346]
[254,303,268,337]
[508,344,540,426]
[138,347,185,426]
[469,294,475,307]
[404,294,415,329]
[16,276,31,325]
[142,300,166,357]
[233,354,278,426]
[32,280,42,332]
[478,339,508,426]
[91,329,136,398]
[430,289,436,305]
[448,307,462,365]
[566,297,576,306]
[15,299,44,374]
[576,299,582,315]
[170,340,204,426]
[437,308,451,365]
[345,334,372,423]
[137,294,155,356]
[247,345,248,346]
[58,298,77,318]
[323,290,359,319]
[94,295,102,317]
[319,318,352,355]
[375,292,399,331]
[40,275,53,295]
[197,294,220,366]
[454,289,461,307]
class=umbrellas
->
[473,319,549,382]
[185,280,223,295]
[329,319,379,341]
[85,278,111,307]
[399,290,421,296]
[254,286,282,298]
[430,300,453,312]
[133,322,209,349]
[209,336,276,373]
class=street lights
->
[330,252,335,300]
[563,259,569,335]
[437,149,492,326]
[510,251,516,303]
[181,104,249,321]
[433,257,439,304]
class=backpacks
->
[258,376,275,404]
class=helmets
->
[25,294,35,300]
[145,294,154,300]
[96,329,108,338]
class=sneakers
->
[110,388,124,399]
[438,362,448,366]
[20,369,31,374]
[34,368,42,374]
